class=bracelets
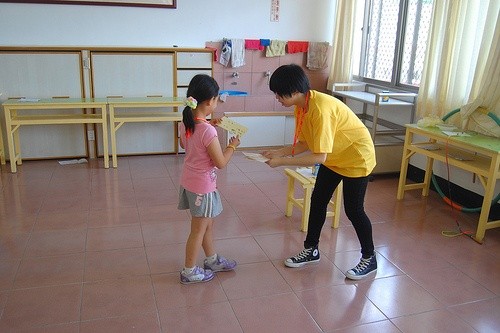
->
[226,145,235,152]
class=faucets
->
[232,72,239,77]
[265,71,271,77]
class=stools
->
[285,166,343,232]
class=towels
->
[218,38,232,68]
[287,40,309,54]
[260,39,271,46]
[230,39,246,68]
[305,41,329,71]
[245,39,265,50]
[265,40,286,57]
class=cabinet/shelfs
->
[332,81,417,178]
[0,42,333,160]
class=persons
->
[178,75,241,284]
[260,64,378,279]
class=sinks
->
[211,111,299,150]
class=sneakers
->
[346,252,377,280]
[284,245,320,267]
[180,266,213,284]
[203,255,236,273]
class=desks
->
[397,123,500,241]
[108,97,189,167]
[3,98,108,172]
